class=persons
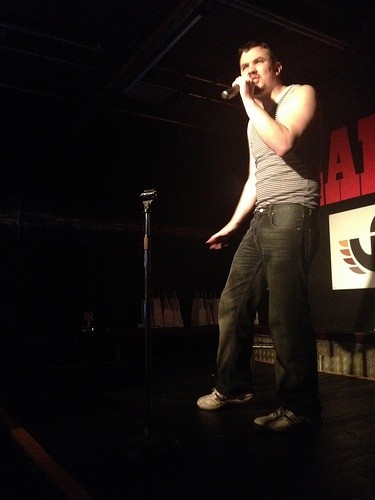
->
[196,39,325,430]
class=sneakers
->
[197,387,254,410]
[253,403,311,429]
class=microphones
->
[222,85,240,100]
